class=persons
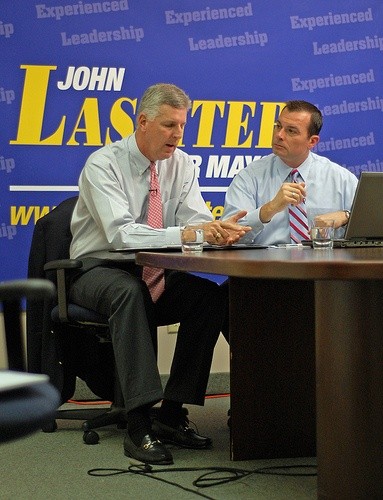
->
[69,84,253,466]
[220,101,360,428]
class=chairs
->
[0,195,190,445]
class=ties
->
[142,161,165,301]
[289,170,310,244]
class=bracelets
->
[343,209,351,214]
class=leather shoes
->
[123,433,173,465]
[152,420,213,450]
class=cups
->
[180,221,204,254]
[309,219,335,250]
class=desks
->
[136,246,383,500]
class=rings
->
[292,192,294,198]
[215,233,220,238]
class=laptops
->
[301,171,383,247]
